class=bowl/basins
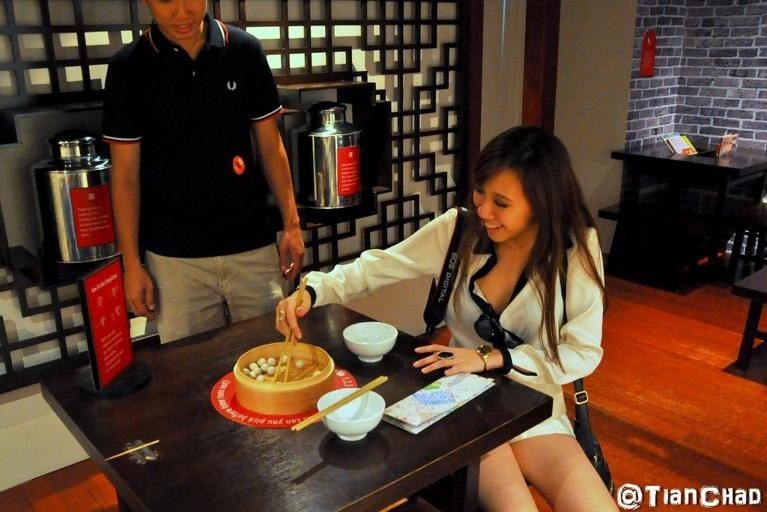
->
[316,388,387,442]
[341,321,399,364]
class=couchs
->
[732,266,767,369]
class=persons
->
[275,125,620,511]
[103,1,304,346]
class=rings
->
[278,311,286,321]
[435,351,455,359]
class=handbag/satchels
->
[574,417,614,497]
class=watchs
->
[472,344,492,372]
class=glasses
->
[475,314,525,348]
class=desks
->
[39,304,552,511]
[610,144,767,296]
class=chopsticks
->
[273,276,309,383]
[289,375,388,433]
